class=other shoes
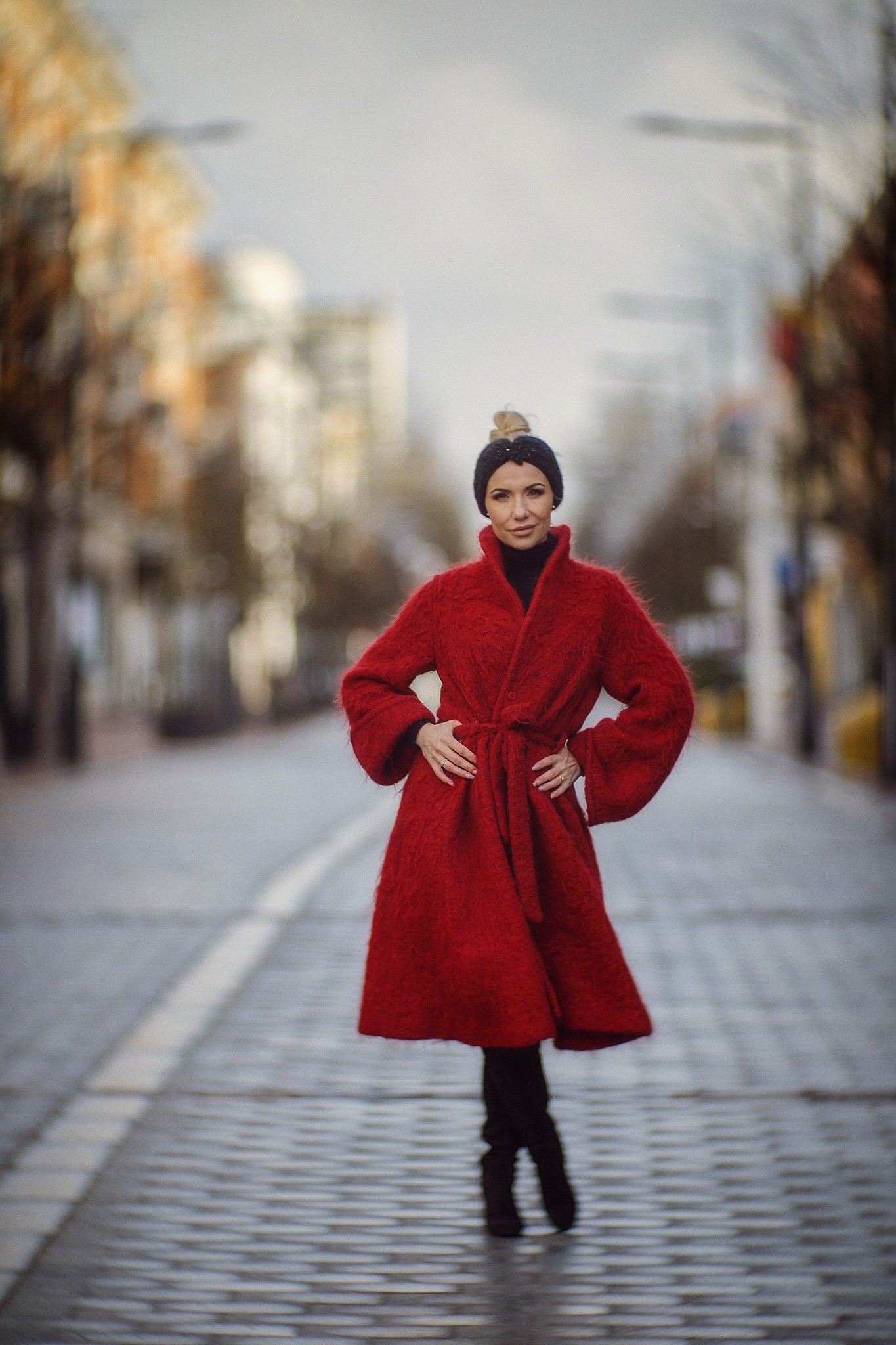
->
[483,1149,522,1235]
[530,1139,576,1231]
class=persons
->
[339,410,696,1237]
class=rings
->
[440,758,448,766]
[558,775,564,781]
